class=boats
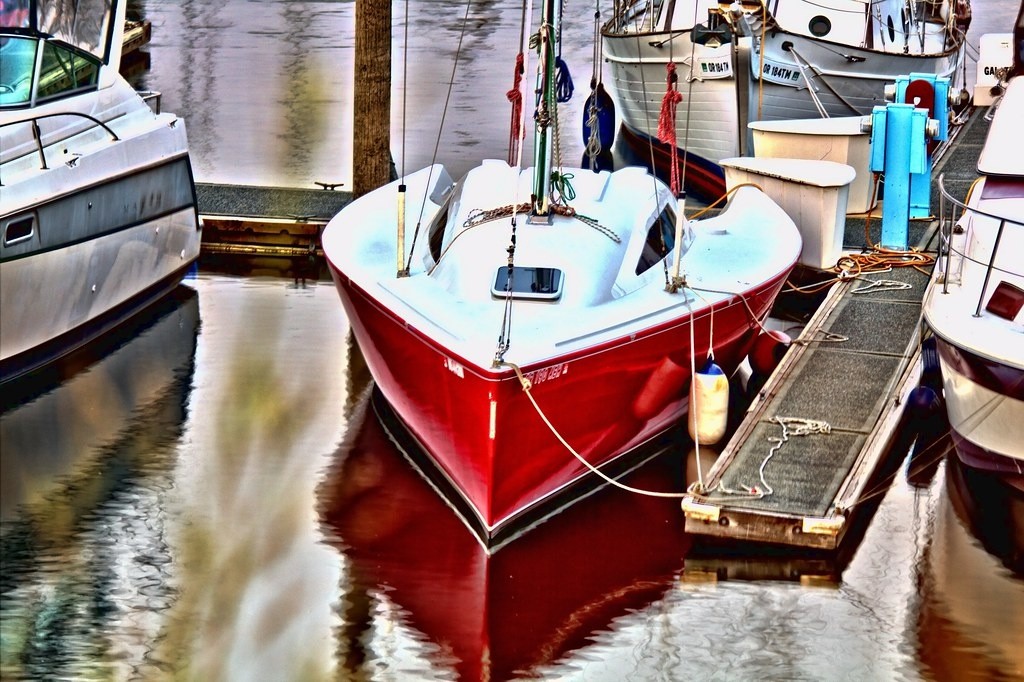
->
[316,374,701,680]
[600,2,971,217]
[2,280,201,588]
[919,66,1024,508]
[917,453,1024,682]
[321,2,803,558]
[0,0,201,368]
